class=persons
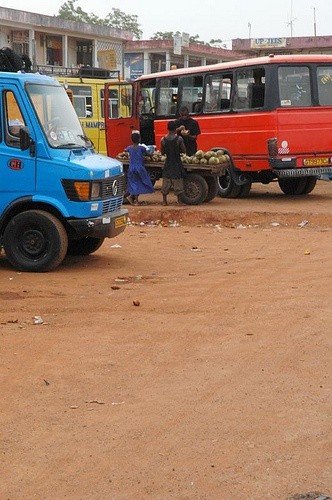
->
[173,106,201,156]
[125,132,155,205]
[160,121,188,206]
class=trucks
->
[0,70,129,272]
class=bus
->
[6,64,156,157]
[103,52,332,206]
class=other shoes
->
[127,197,134,206]
[135,196,140,204]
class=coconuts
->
[192,150,230,165]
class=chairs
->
[169,99,231,114]
